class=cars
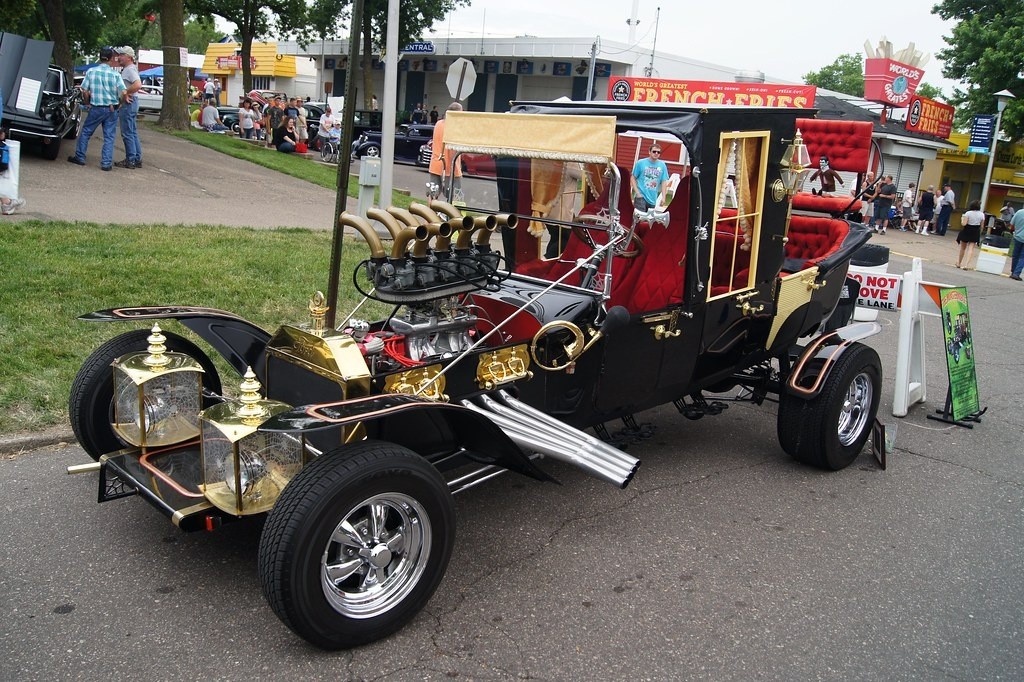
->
[137,85,163,113]
[301,101,382,152]
[351,123,435,168]
[0,31,84,160]
[66,98,891,652]
[217,89,289,137]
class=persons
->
[850,172,897,234]
[630,143,669,213]
[237,95,311,147]
[428,102,462,207]
[999,202,1015,224]
[114,45,142,169]
[274,116,299,153]
[545,161,584,259]
[187,86,229,131]
[142,77,160,94]
[1009,208,1024,281]
[930,189,944,233]
[372,94,379,111]
[430,105,438,123]
[410,103,423,124]
[422,104,429,124]
[318,107,357,156]
[934,184,956,237]
[67,47,127,171]
[915,185,937,235]
[201,78,221,107]
[956,200,985,270]
[897,182,916,232]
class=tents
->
[139,66,164,90]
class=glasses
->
[651,150,662,154]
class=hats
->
[117,46,134,57]
[943,183,951,187]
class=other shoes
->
[102,166,112,170]
[133,159,142,168]
[1010,273,1022,280]
[68,156,85,165]
[873,225,943,235]
[114,159,135,168]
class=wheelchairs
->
[320,132,354,165]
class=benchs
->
[710,207,850,300]
[791,117,874,218]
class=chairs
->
[607,175,690,316]
[546,167,638,293]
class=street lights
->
[967,89,1016,270]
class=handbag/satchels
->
[296,142,307,153]
